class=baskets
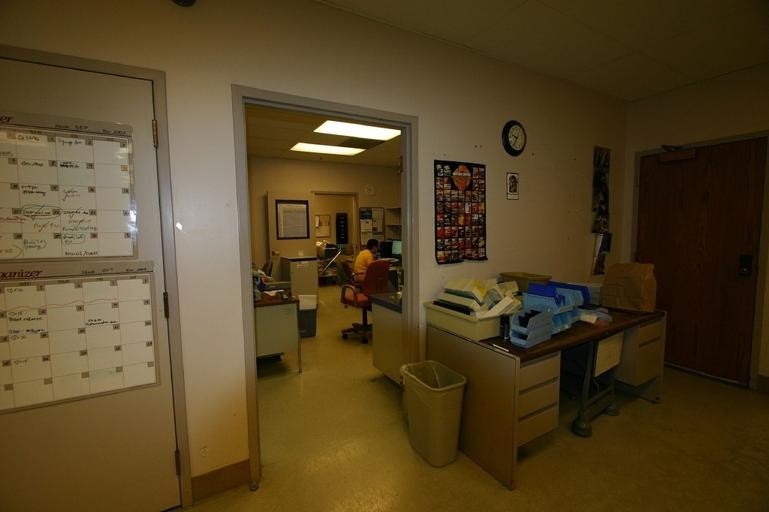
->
[500,271,552,292]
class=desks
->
[254,289,302,374]
[424,304,667,490]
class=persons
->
[354,239,379,282]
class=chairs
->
[564,328,628,438]
[340,258,390,344]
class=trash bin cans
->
[400,360,467,467]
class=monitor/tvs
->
[392,240,403,255]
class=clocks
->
[502,119,527,157]
